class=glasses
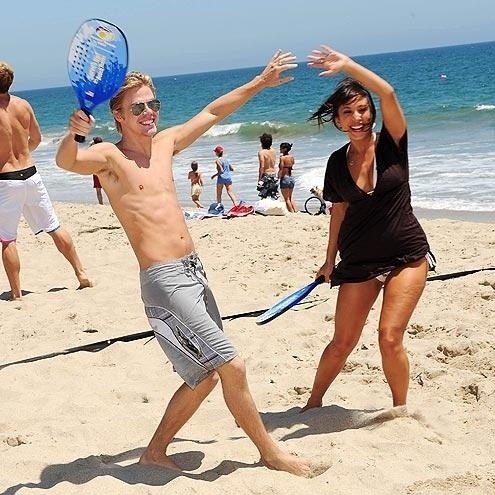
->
[119,98,160,117]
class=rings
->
[277,60,284,65]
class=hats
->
[208,203,224,216]
[213,145,224,154]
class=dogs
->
[310,185,325,203]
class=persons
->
[57,49,330,477]
[299,43,438,412]
[187,160,205,209]
[210,145,239,207]
[0,61,95,301]
[255,133,280,201]
[276,142,297,213]
[88,136,104,206]
